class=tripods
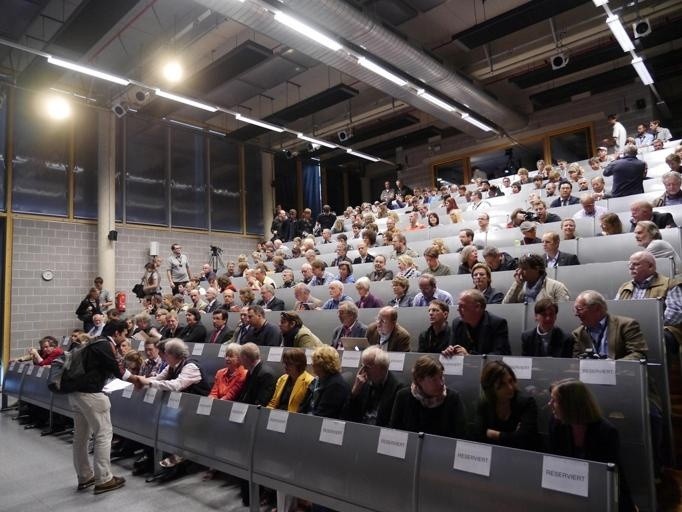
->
[207,254,226,273]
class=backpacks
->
[47,336,108,393]
[133,284,146,298]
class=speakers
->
[110,230,117,240]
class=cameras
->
[577,348,601,359]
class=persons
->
[8,108,681,510]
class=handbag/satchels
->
[77,311,93,322]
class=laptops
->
[340,336,370,350]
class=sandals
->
[159,453,188,467]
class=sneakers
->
[93,474,125,494]
[111,447,134,457]
[76,475,95,489]
[133,453,152,469]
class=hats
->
[520,221,537,232]
[596,146,608,153]
